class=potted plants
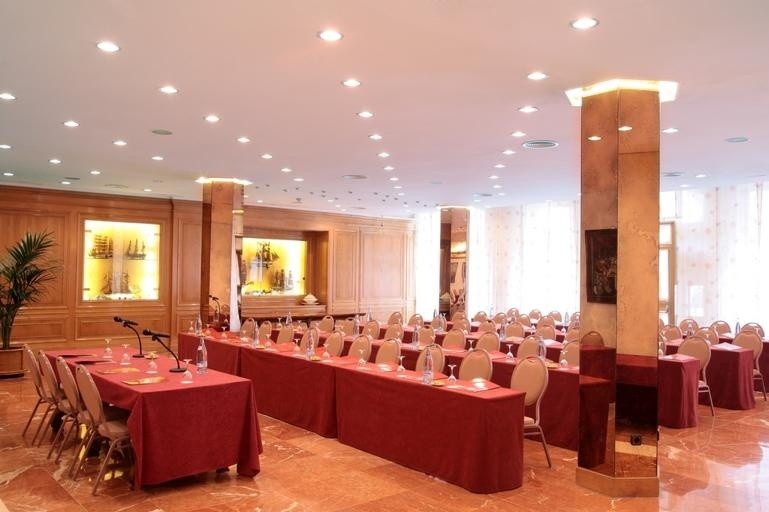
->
[1,225,66,377]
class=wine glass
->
[397,318,401,324]
[297,320,302,328]
[528,328,535,339]
[429,335,436,349]
[205,324,210,336]
[396,332,401,345]
[463,324,468,335]
[448,364,457,384]
[322,343,329,362]
[563,333,569,346]
[120,343,130,365]
[506,344,513,361]
[560,351,568,367]
[357,349,367,368]
[538,312,542,320]
[417,319,421,330]
[264,333,272,351]
[366,328,373,341]
[221,326,227,340]
[468,340,475,353]
[294,339,300,357]
[397,355,406,377]
[147,350,158,373]
[277,318,282,327]
[189,321,193,331]
[240,330,247,343]
[104,338,112,359]
[182,359,193,382]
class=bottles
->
[423,346,433,384]
[253,320,259,346]
[686,322,695,337]
[352,314,359,341]
[366,305,372,320]
[194,312,202,336]
[500,320,506,339]
[489,303,495,319]
[537,336,546,361]
[412,325,419,347]
[286,312,291,325]
[565,311,569,323]
[306,330,315,360]
[439,313,444,332]
[196,335,208,375]
[433,308,437,319]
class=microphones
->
[143,329,187,372]
[114,316,148,357]
[208,295,220,322]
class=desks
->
[616,353,657,388]
[425,321,756,410]
[359,323,700,429]
[268,323,579,452]
[580,375,612,468]
[178,328,528,495]
[486,318,769,392]
[580,345,615,403]
[32,345,264,493]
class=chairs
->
[473,310,487,321]
[375,339,401,365]
[475,331,501,351]
[549,310,562,322]
[415,343,446,373]
[300,328,319,349]
[293,322,307,328]
[324,330,344,356]
[451,318,471,332]
[676,336,716,417]
[240,318,255,339]
[276,323,295,343]
[450,309,467,322]
[537,316,557,327]
[458,348,493,381]
[73,363,134,495]
[581,331,604,344]
[342,317,356,336]
[21,343,49,444]
[348,335,372,362]
[443,315,448,329]
[677,320,697,334]
[492,312,506,322]
[517,314,531,327]
[570,312,580,321]
[698,326,719,344]
[510,354,551,467]
[713,321,731,335]
[259,321,272,338]
[408,313,424,326]
[47,355,79,463]
[563,330,579,339]
[478,319,497,331]
[568,320,580,329]
[536,325,556,339]
[318,314,334,333]
[516,335,547,357]
[32,349,59,447]
[384,325,405,342]
[741,321,764,337]
[506,307,518,321]
[661,324,682,340]
[560,340,579,364]
[658,318,665,332]
[731,332,768,403]
[442,327,467,349]
[505,321,525,337]
[432,315,443,329]
[387,312,403,325]
[530,309,542,320]
[418,325,436,346]
[362,320,380,338]
